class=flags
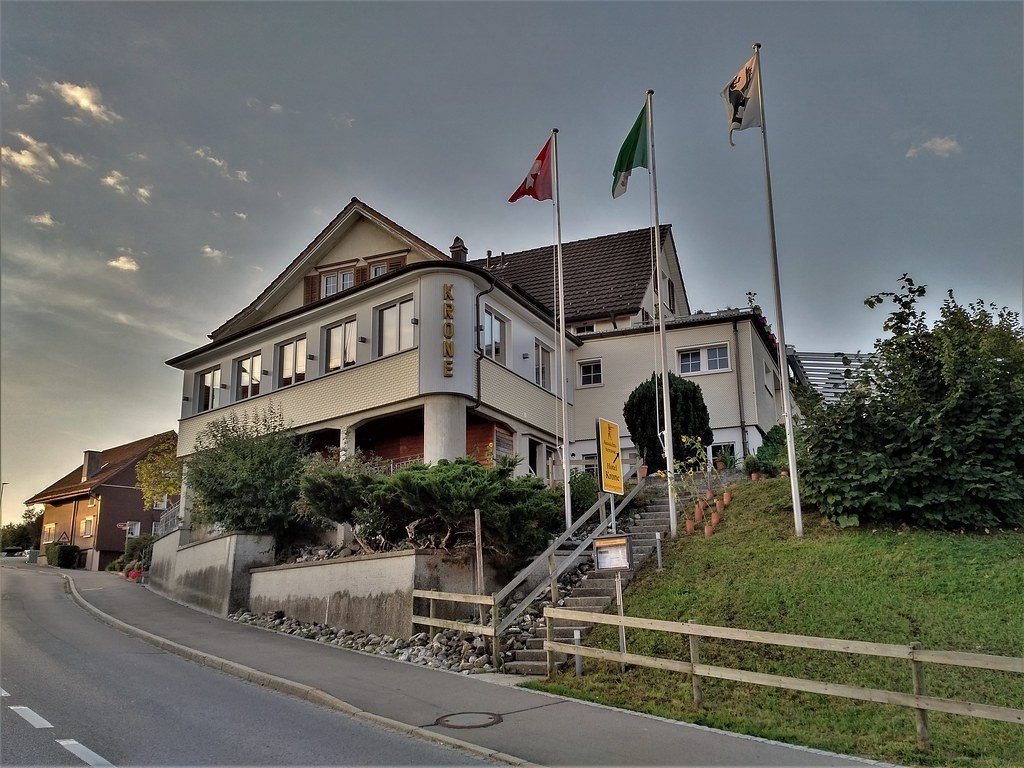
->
[612,98,649,198]
[719,55,763,148]
[508,132,553,204]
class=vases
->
[695,508,702,521]
[712,512,720,526]
[724,492,730,506]
[705,526,713,539]
[686,519,695,534]
[699,500,706,510]
[716,501,724,512]
[707,490,714,500]
[752,473,759,480]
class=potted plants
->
[753,305,762,316]
[764,324,772,333]
[635,442,648,477]
[769,333,777,343]
[774,343,779,349]
[714,443,732,469]
[772,460,789,478]
[760,316,767,325]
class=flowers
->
[657,435,726,524]
